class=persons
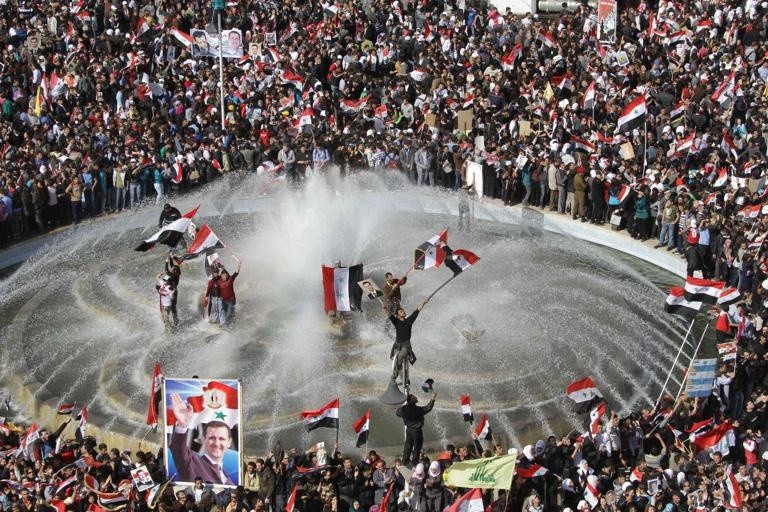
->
[393,392,438,469]
[0,407,767,511]
[327,259,349,324]
[379,271,408,326]
[1,0,119,248]
[361,281,384,301]
[121,1,601,226]
[155,252,241,335]
[382,299,428,388]
[600,1,767,406]
[157,202,188,253]
[167,389,238,486]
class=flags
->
[564,377,604,414]
[203,248,222,277]
[73,404,90,442]
[410,230,449,269]
[439,240,479,275]
[457,391,476,424]
[56,401,76,416]
[297,397,340,435]
[132,204,200,253]
[145,361,165,436]
[320,263,366,313]
[167,224,227,264]
